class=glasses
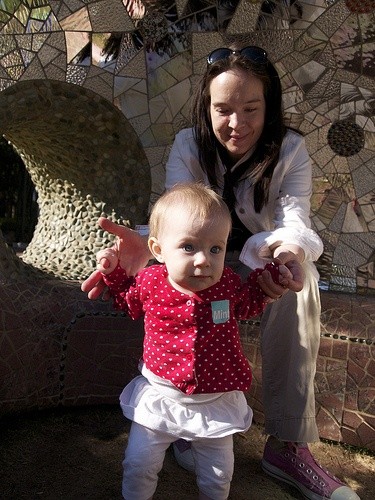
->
[206,45,268,68]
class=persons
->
[80,45,360,500]
[95,182,292,500]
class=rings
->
[278,294,283,299]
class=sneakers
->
[261,435,361,500]
[174,438,196,472]
